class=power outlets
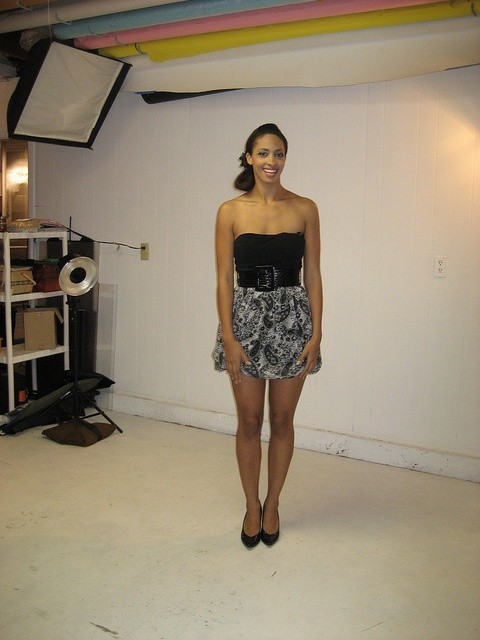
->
[140,243,150,260]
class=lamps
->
[6,36,134,149]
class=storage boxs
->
[0,265,37,294]
[13,307,26,340]
[23,307,64,350]
[33,264,62,293]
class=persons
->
[212,122,323,548]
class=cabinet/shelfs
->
[0,230,71,423]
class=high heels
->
[241,505,262,546]
[261,502,279,546]
